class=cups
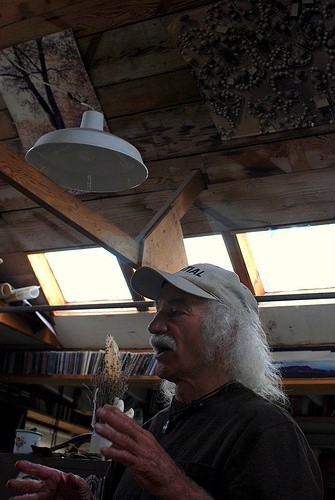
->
[90,429,113,461]
[13,429,43,454]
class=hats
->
[130,264,259,314]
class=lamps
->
[26,111,150,194]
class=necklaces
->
[162,400,214,435]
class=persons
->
[5,264,325,500]
